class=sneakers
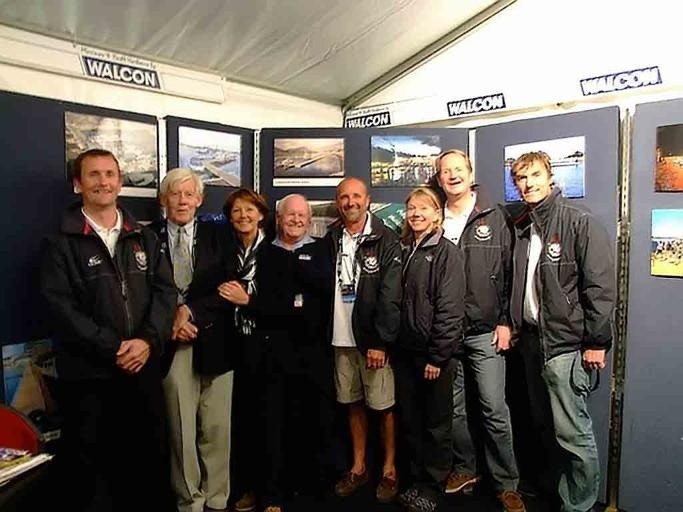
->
[500,490,525,511]
[444,472,476,494]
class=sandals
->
[235,493,257,510]
[375,474,396,500]
[333,469,367,494]
[264,505,281,512]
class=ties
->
[172,227,192,290]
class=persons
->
[137,166,237,512]
[313,175,405,501]
[428,148,528,512]
[504,151,616,512]
[268,193,333,503]
[398,187,464,489]
[39,148,177,512]
[217,187,283,511]
